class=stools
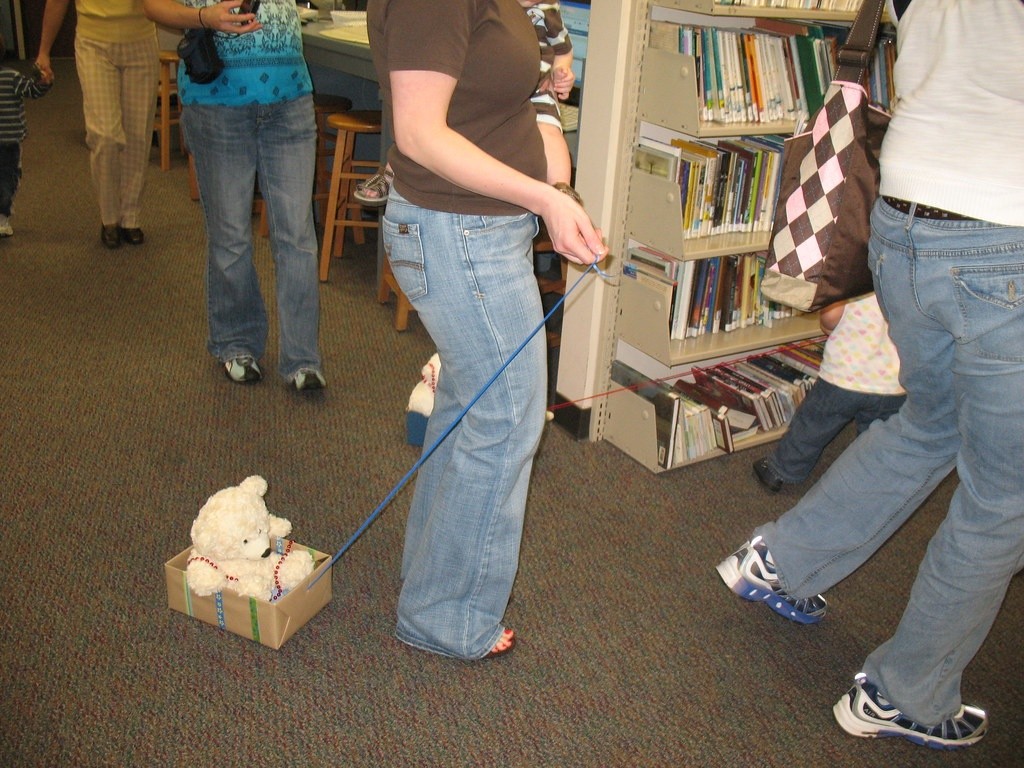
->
[150,50,567,336]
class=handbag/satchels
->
[760,1,914,312]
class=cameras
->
[238,0,260,25]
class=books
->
[626,242,808,340]
[676,22,898,123]
[632,130,790,239]
[612,333,829,470]
[715,0,888,13]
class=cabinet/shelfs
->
[546,0,900,474]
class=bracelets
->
[550,182,584,206]
[198,6,208,29]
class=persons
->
[36,0,162,249]
[0,45,54,237]
[138,0,331,398]
[354,2,610,661]
[751,290,907,494]
[713,0,1024,746]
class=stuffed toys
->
[401,349,447,422]
[178,470,320,604]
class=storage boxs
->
[164,537,333,651]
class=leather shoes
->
[118,225,142,245]
[101,221,118,249]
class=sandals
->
[552,180,579,206]
[354,170,396,203]
[487,627,517,657]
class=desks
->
[298,20,583,273]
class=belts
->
[882,194,977,221]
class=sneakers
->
[293,366,325,394]
[0,214,14,237]
[225,355,262,382]
[833,673,989,748]
[714,535,828,623]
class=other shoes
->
[754,456,784,494]
